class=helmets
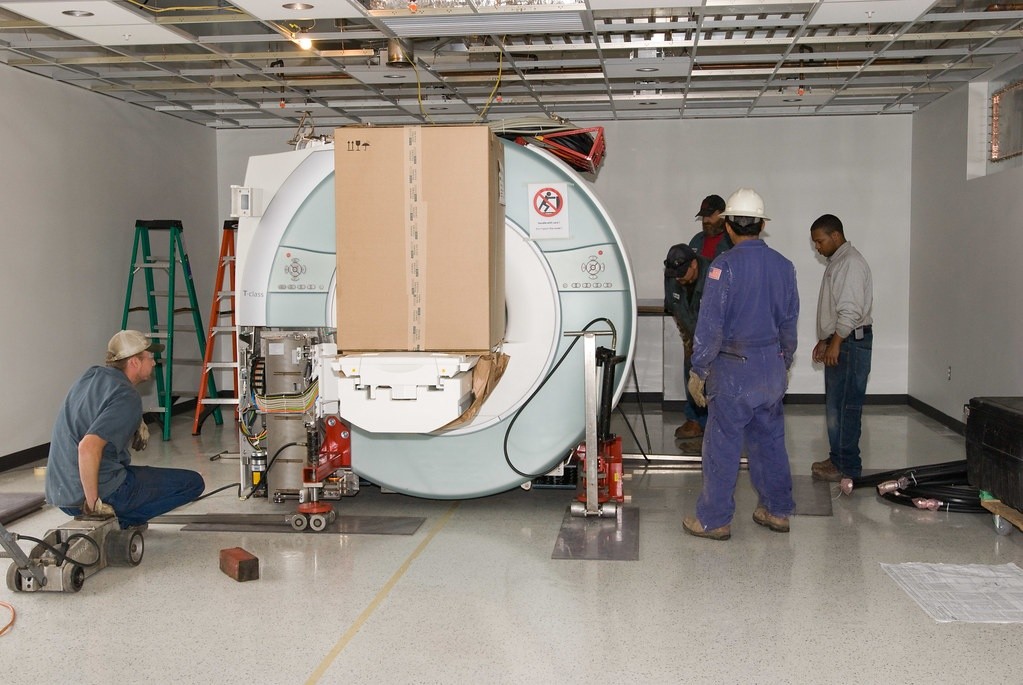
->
[718,184,772,221]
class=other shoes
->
[675,421,706,437]
[125,521,147,533]
[680,437,705,454]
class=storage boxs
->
[332,124,505,354]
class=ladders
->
[192,220,239,435]
[121,219,223,441]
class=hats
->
[107,330,166,364]
[694,195,725,217]
[662,245,696,278]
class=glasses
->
[664,257,692,269]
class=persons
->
[44,328,204,529]
[686,194,734,260]
[662,243,713,439]
[811,213,874,478]
[681,188,800,540]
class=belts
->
[852,328,872,334]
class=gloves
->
[82,495,117,518]
[688,370,708,409]
[131,419,149,451]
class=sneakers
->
[812,458,862,482]
[684,513,730,540]
[753,506,789,533]
